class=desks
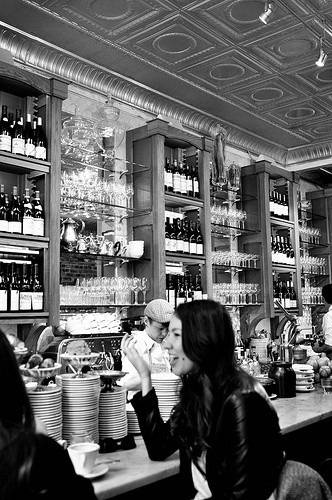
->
[91,383,332,500]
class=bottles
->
[268,191,299,310]
[166,272,209,310]
[59,92,122,163]
[268,361,296,398]
[0,260,44,313]
[1,93,47,238]
[233,345,261,378]
[163,155,205,256]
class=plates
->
[55,371,99,446]
[77,460,110,479]
[21,377,65,450]
[291,365,315,391]
[99,385,141,439]
[269,394,277,399]
[150,373,183,423]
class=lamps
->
[315,46,331,68]
[259,0,278,24]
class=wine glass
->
[297,196,329,305]
[59,164,136,223]
[210,203,263,307]
[59,277,150,305]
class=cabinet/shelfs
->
[1,59,332,325]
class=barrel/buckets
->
[249,338,268,363]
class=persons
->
[121,299,284,500]
[311,284,332,360]
[0,329,99,500]
[121,298,176,400]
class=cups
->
[128,240,144,258]
[70,432,93,446]
[68,443,100,475]
[57,311,122,334]
[321,379,332,395]
[279,344,306,363]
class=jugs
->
[58,216,127,256]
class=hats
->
[144,299,175,323]
